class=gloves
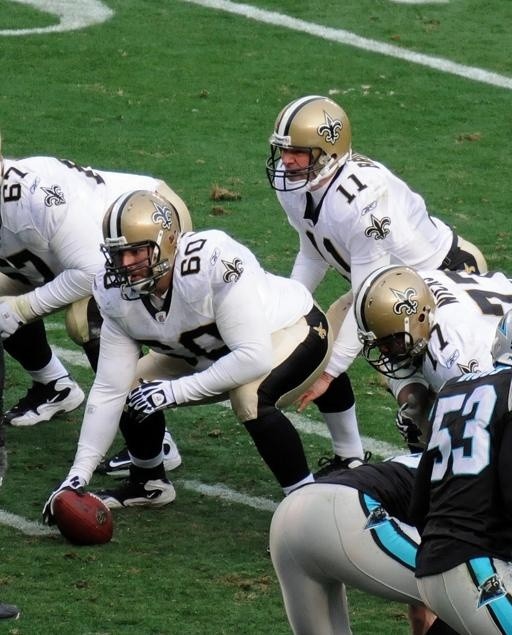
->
[123,378,176,423]
[41,475,86,526]
[0,295,27,337]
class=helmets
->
[354,264,435,379]
[100,190,181,301]
[264,95,352,191]
[491,309,512,367]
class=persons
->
[353,264,512,454]
[410,308,512,635]
[265,95,489,413]
[269,452,437,635]
[43,189,334,553]
[0,156,192,476]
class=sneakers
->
[314,451,372,479]
[93,477,176,508]
[94,427,181,476]
[4,372,84,426]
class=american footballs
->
[51,489,113,543]
